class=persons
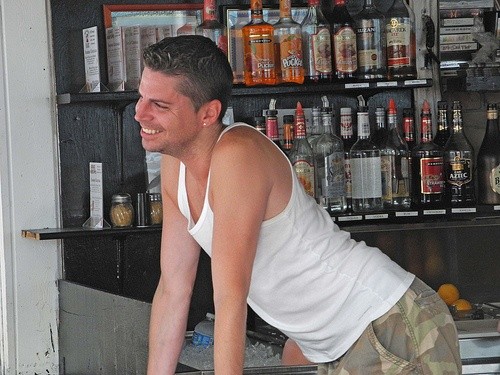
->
[134,35,463,375]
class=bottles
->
[190,312,215,349]
[148,191,163,224]
[186,0,500,215]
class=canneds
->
[109,192,134,228]
[146,193,163,224]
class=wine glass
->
[109,192,136,227]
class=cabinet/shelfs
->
[22,0,500,240]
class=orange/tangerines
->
[438,283,473,310]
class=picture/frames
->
[102,4,204,29]
[219,3,309,87]
